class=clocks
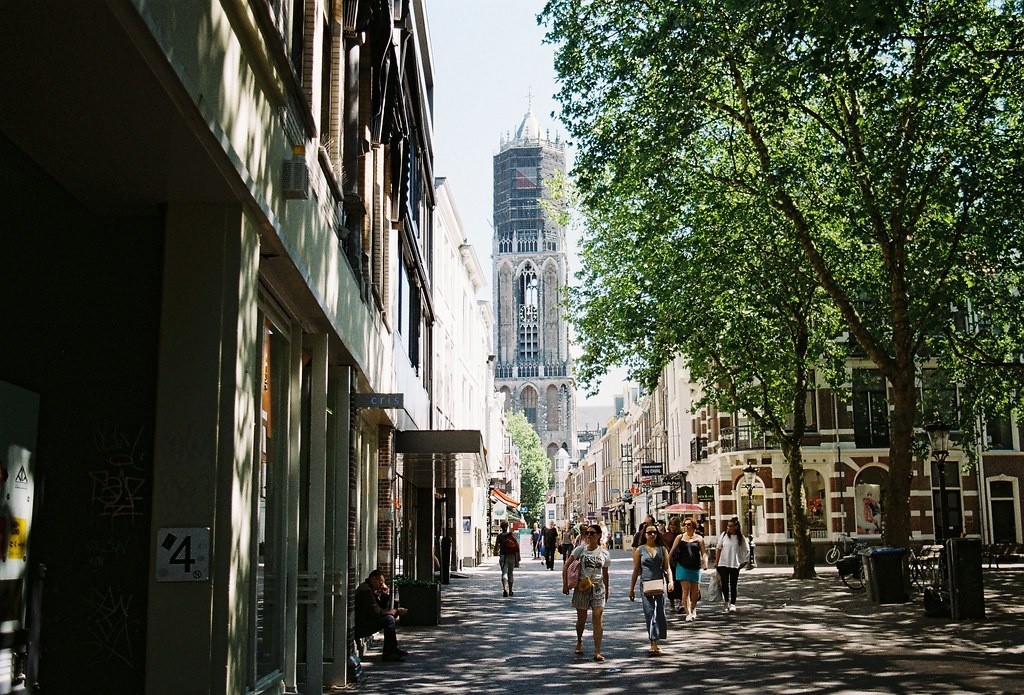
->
[519,304,538,322]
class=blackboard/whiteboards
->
[613,530,623,546]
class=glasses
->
[585,531,597,535]
[644,531,657,535]
[684,523,693,527]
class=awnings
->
[491,488,520,507]
[506,510,520,520]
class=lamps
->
[633,478,642,489]
[587,501,593,508]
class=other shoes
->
[510,592,513,596]
[729,604,736,612]
[382,653,405,661]
[594,654,605,661]
[550,566,553,571]
[691,607,696,618]
[394,648,408,655]
[575,640,582,653]
[686,613,692,622]
[649,646,661,654]
[541,562,544,565]
[504,591,508,597]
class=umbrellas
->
[662,504,707,521]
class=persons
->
[542,519,558,571]
[629,523,674,654]
[562,525,610,662]
[537,526,546,565]
[631,516,704,614]
[713,517,750,612]
[493,521,521,598]
[669,517,708,621]
[557,520,608,564]
[530,522,540,560]
[355,570,409,662]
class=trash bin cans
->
[857,547,911,603]
[945,538,986,622]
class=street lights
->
[588,500,593,526]
[923,410,950,558]
[741,461,759,570]
[488,478,496,553]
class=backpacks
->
[501,532,518,554]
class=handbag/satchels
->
[567,546,585,588]
[558,544,562,554]
[643,579,664,596]
[579,576,593,591]
[707,568,723,601]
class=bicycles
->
[824,531,866,591]
[907,546,942,590]
